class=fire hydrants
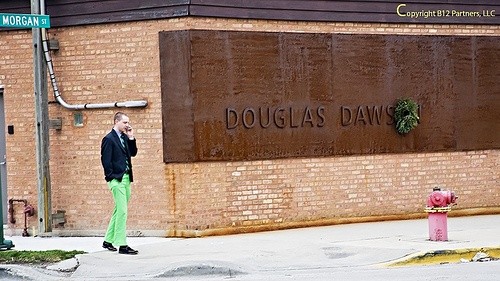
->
[424,187,458,241]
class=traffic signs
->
[0,13,51,28]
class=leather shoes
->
[102,240,117,250]
[119,244,138,254]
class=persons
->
[101,112,138,255]
